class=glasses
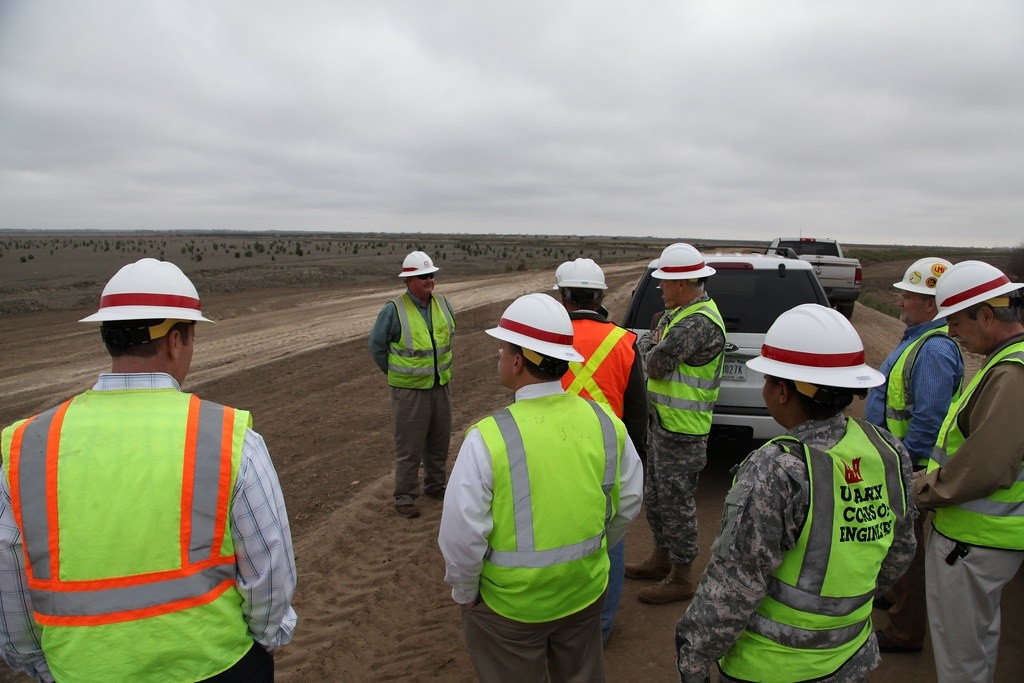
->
[417,273,435,280]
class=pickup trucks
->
[765,237,863,320]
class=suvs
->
[622,247,832,446]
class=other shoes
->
[874,595,896,612]
[429,488,447,499]
[394,501,419,518]
[873,632,920,654]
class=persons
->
[0,258,297,683]
[369,251,456,519]
[438,294,644,683]
[909,260,1024,683]
[623,243,727,604]
[866,257,964,655]
[546,259,646,652]
[675,303,918,683]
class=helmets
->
[77,257,215,325]
[484,292,586,362]
[651,246,715,279]
[398,251,440,278]
[559,255,608,293]
[746,302,886,388]
[932,261,1024,322]
[553,261,574,291]
[892,256,953,295]
[648,257,660,269]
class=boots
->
[623,554,672,581]
[638,564,693,604]
